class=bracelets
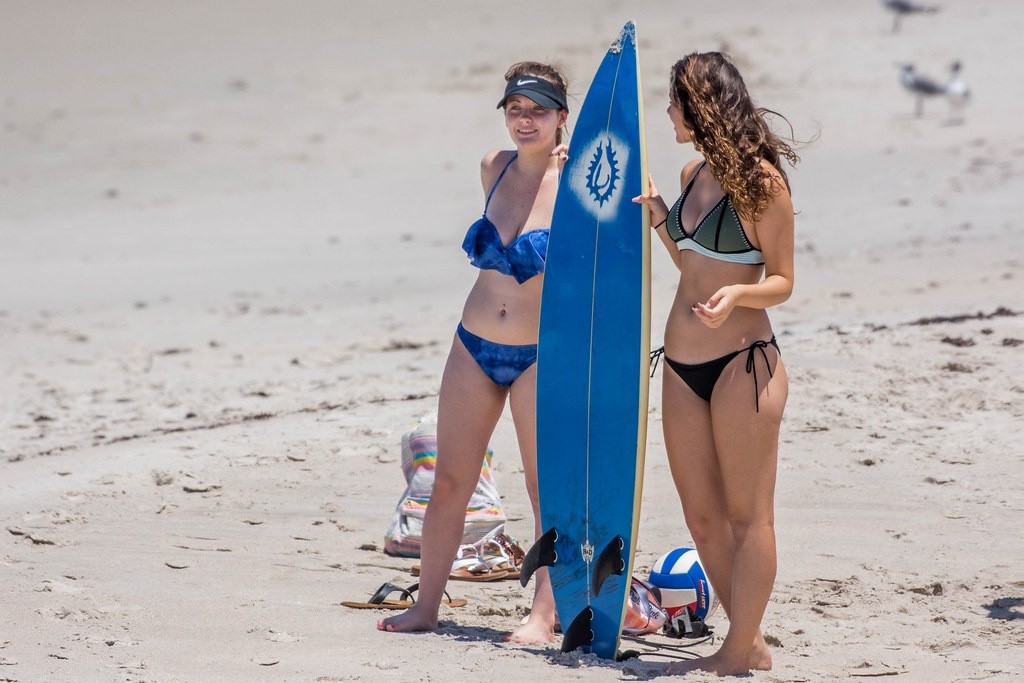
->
[654,217,666,230]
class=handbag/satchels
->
[384,410,506,558]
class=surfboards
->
[519,19,654,666]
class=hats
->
[496,75,569,113]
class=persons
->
[632,51,795,678]
[377,61,568,643]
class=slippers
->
[409,531,526,581]
[338,583,467,609]
